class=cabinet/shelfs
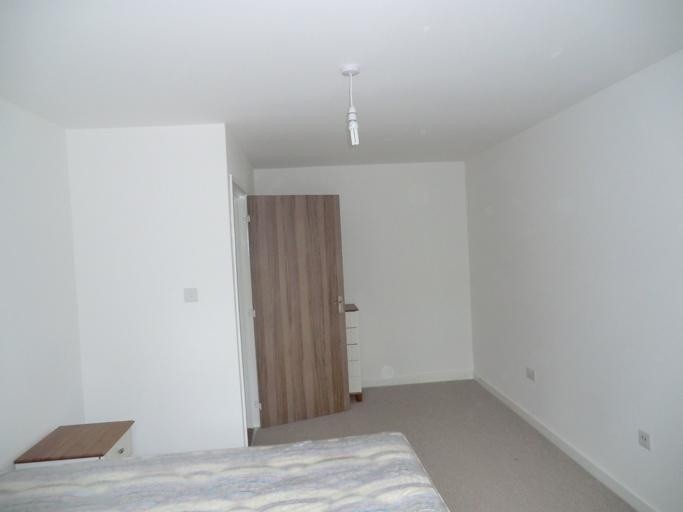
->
[345,303,363,402]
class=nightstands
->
[14,420,135,472]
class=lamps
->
[341,63,360,146]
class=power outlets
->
[636,429,651,452]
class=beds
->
[0,431,450,512]
